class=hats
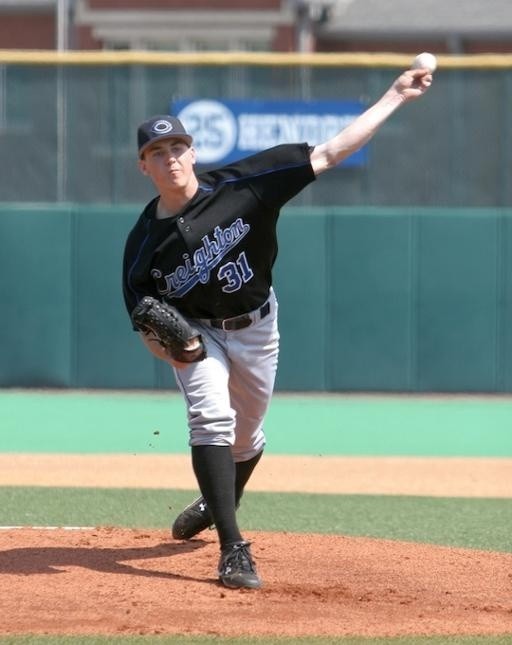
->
[137,114,192,157]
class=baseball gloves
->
[129,297,208,366]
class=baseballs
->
[414,54,436,75]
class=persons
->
[120,67,433,587]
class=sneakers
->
[173,494,240,540]
[218,541,260,589]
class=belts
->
[211,304,269,331]
